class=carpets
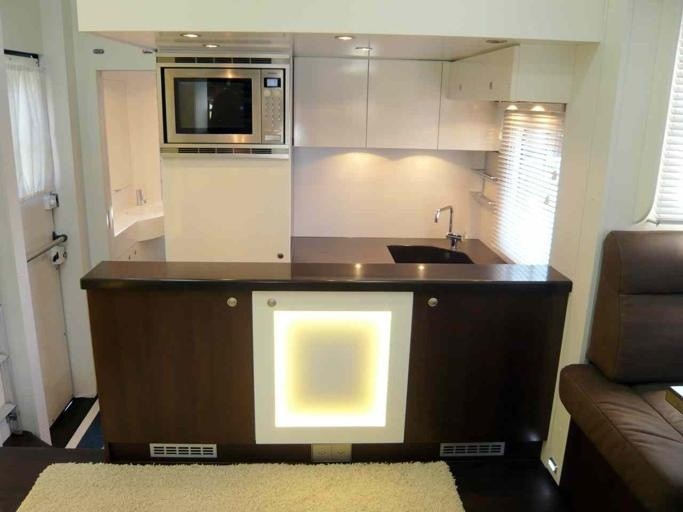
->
[14,460,467,512]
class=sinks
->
[127,204,164,242]
[387,244,474,262]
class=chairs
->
[559,231,683,512]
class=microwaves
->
[163,65,286,146]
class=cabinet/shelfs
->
[293,55,503,152]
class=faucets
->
[435,204,454,235]
[136,188,148,206]
